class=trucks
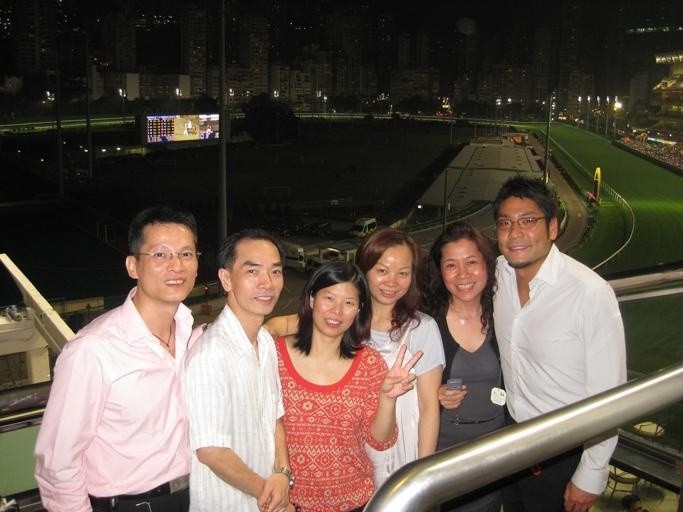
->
[348,215,378,239]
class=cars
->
[436,110,447,117]
[442,101,448,109]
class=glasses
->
[138,249,202,264]
[495,216,548,232]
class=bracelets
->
[270,466,295,487]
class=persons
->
[177,226,299,512]
[412,219,511,512]
[484,174,631,512]
[29,204,212,512]
[256,227,448,489]
[620,493,650,511]
[265,259,425,512]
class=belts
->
[89,475,189,509]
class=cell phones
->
[447,379,463,390]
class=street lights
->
[117,86,128,126]
[227,84,335,119]
[173,86,183,114]
[44,91,57,130]
[575,93,620,138]
[495,96,501,126]
[507,97,512,125]
